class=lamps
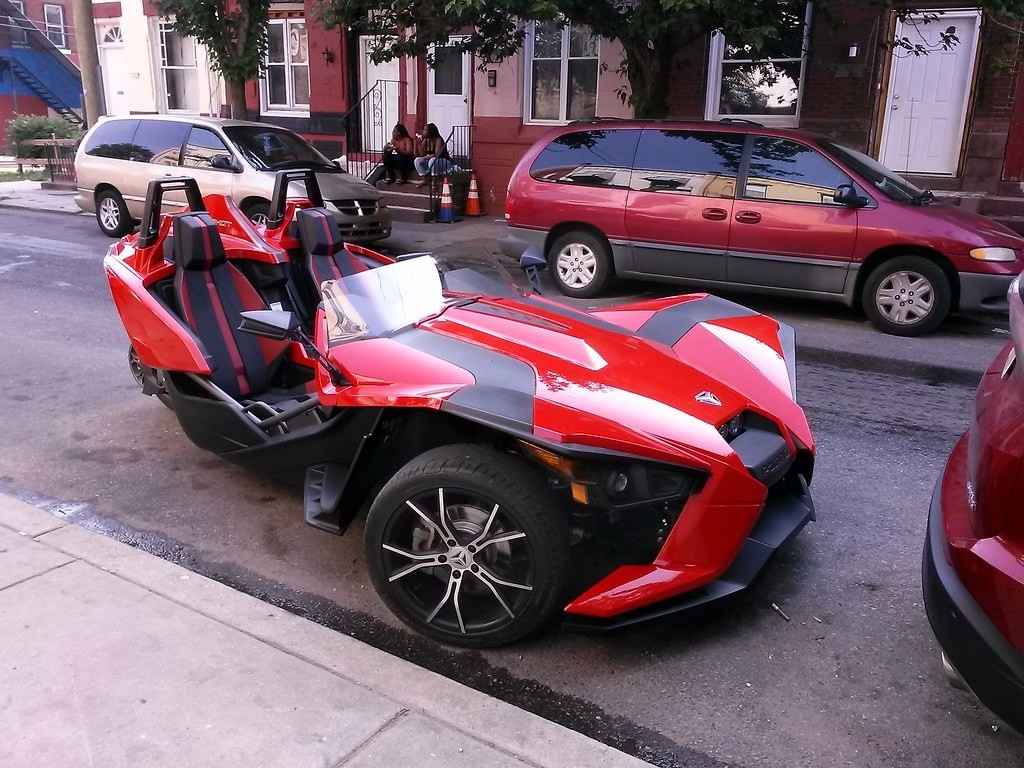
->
[849,43,861,58]
[322,45,332,66]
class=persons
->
[383,124,415,184]
[414,123,451,188]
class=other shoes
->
[383,177,394,184]
[416,179,428,188]
[431,179,439,190]
[395,177,406,184]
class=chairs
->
[296,207,370,300]
[172,210,321,414]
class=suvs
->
[498,116,1024,336]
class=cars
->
[103,167,815,650]
[922,267,1024,741]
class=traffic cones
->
[461,173,486,216]
[435,178,463,223]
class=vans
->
[73,114,392,243]
